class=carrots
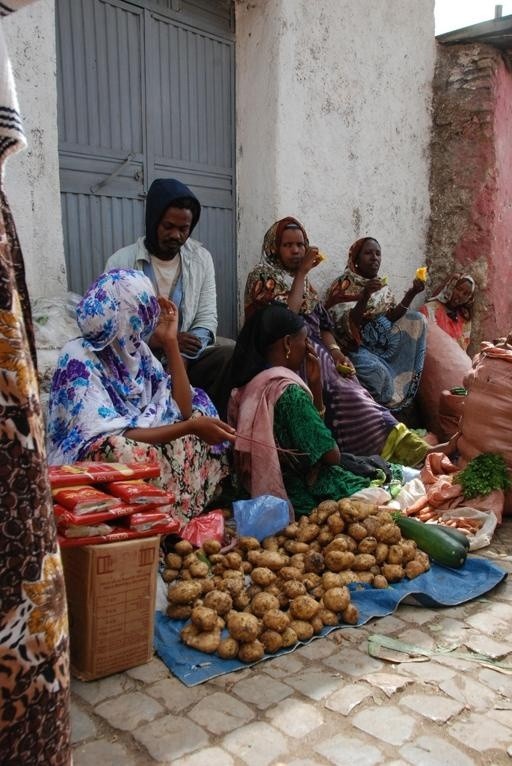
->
[400,496,484,536]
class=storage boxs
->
[62,535,160,683]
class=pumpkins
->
[395,517,471,570]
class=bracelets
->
[325,343,340,351]
[398,302,409,311]
[316,406,328,416]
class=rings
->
[316,356,321,360]
[168,310,175,316]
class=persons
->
[104,178,236,378]
[244,216,461,461]
[328,237,428,417]
[218,300,377,511]
[50,270,236,523]
[417,273,476,352]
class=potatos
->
[162,498,431,663]
